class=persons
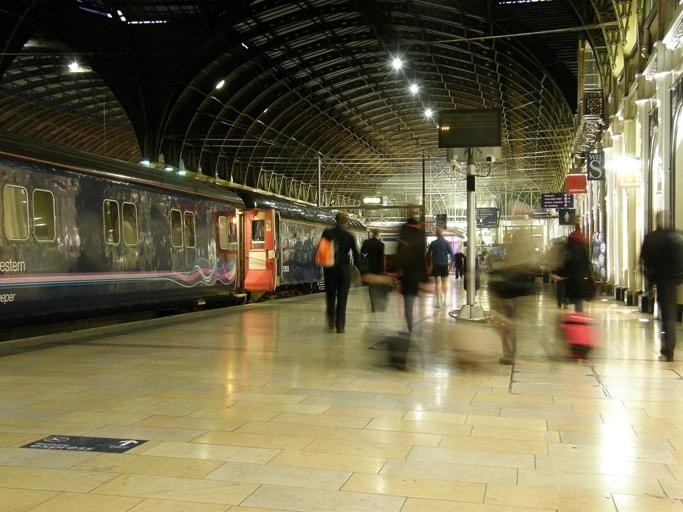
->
[394,217,432,335]
[427,228,455,308]
[454,247,466,279]
[361,229,384,312]
[320,213,360,334]
[638,210,683,363]
[549,216,597,313]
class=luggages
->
[558,310,605,352]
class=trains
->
[0,136,369,320]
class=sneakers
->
[435,301,447,308]
[326,315,344,334]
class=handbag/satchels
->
[315,237,335,267]
